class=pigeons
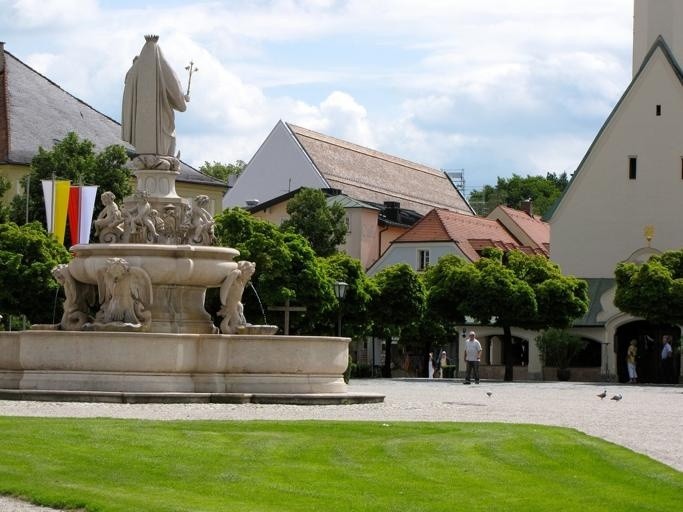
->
[610,392,623,403]
[486,392,492,397]
[596,390,607,400]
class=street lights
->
[333,278,348,337]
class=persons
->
[91,190,120,236]
[120,33,192,155]
[660,334,673,384]
[121,188,161,238]
[462,330,482,385]
[624,339,638,384]
[191,194,217,242]
[437,350,451,377]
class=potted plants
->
[532,324,590,381]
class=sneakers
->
[463,379,480,384]
